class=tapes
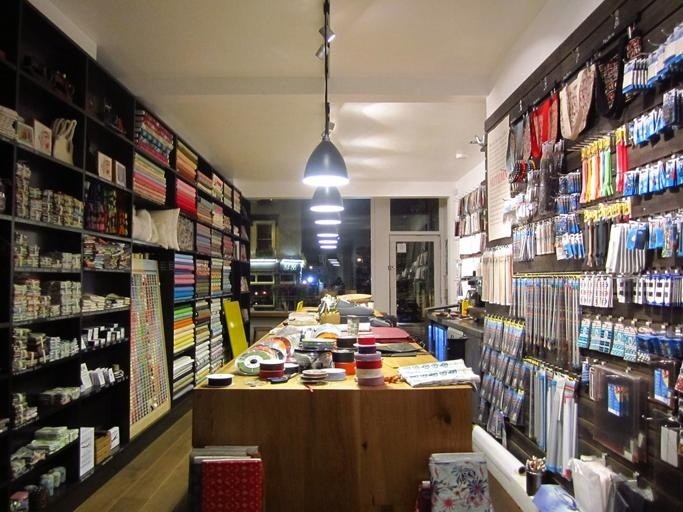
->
[258,361,300,381]
[320,337,385,386]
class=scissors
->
[266,372,297,383]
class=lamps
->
[315,227,339,249]
[302,0,350,226]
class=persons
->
[331,277,346,295]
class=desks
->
[189,306,473,512]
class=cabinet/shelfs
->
[0,0,253,512]
[427,309,483,425]
[482,1,682,512]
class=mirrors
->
[243,195,374,316]
